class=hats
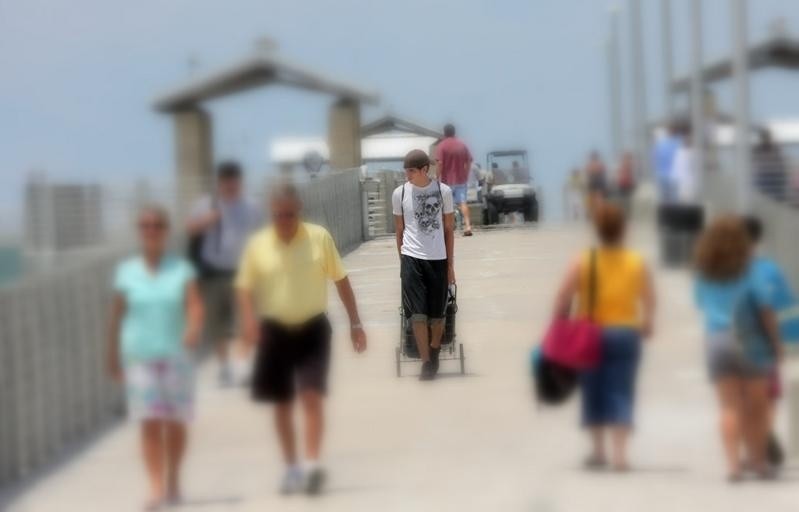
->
[404,149,429,169]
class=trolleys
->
[396,277,466,377]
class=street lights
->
[303,149,324,180]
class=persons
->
[184,159,268,388]
[552,204,655,473]
[389,148,455,382]
[732,216,798,470]
[692,212,783,486]
[108,205,205,510]
[434,124,475,235]
[562,117,786,267]
[233,182,368,498]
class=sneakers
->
[419,361,434,380]
[429,345,442,372]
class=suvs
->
[481,148,539,225]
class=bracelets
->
[350,322,365,332]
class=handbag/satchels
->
[535,352,583,403]
[541,317,601,369]
